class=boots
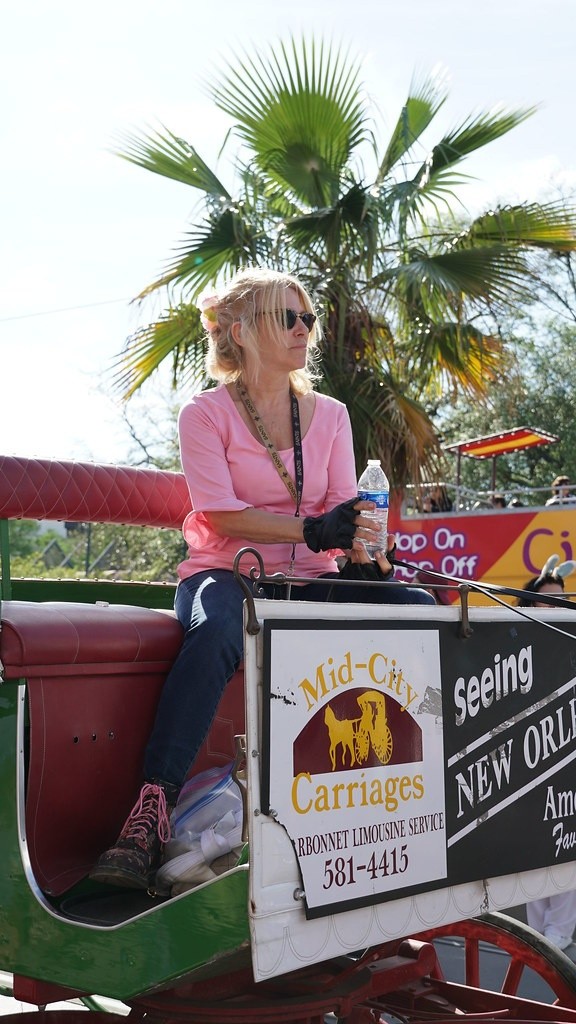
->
[88,782,171,891]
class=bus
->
[386,423,576,607]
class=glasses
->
[253,308,316,333]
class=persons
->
[518,554,576,608]
[526,889,576,963]
[421,487,452,513]
[546,476,576,507]
[488,495,522,509]
[92,270,437,888]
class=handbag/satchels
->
[154,809,245,897]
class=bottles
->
[353,459,389,560]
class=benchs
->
[0,457,244,897]
